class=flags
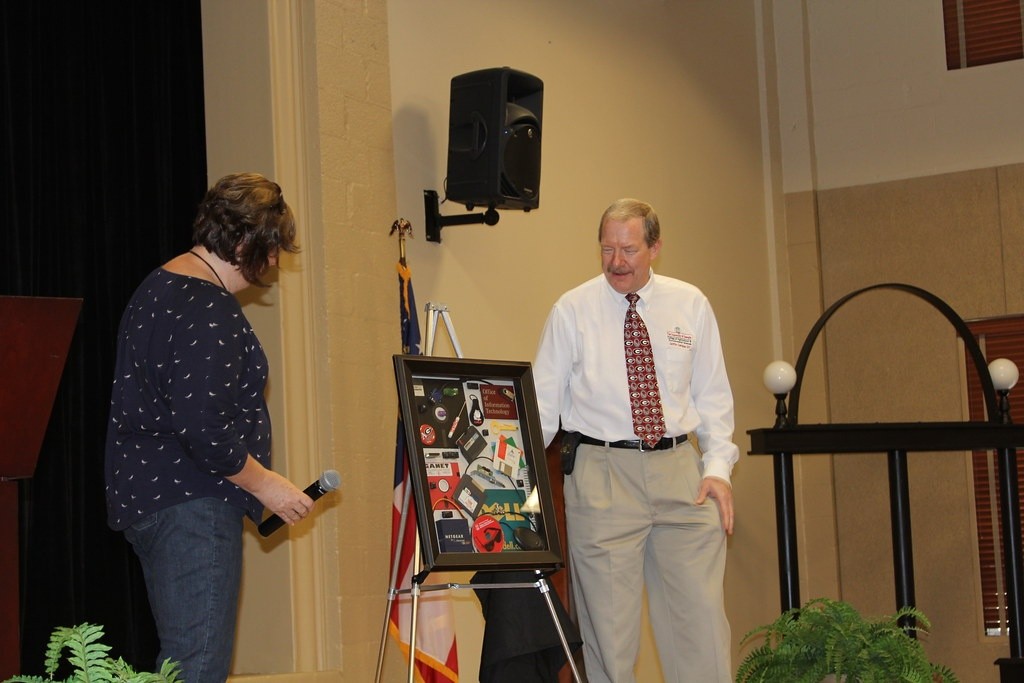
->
[386,256,461,683]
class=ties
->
[625,293,667,449]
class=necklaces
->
[186,248,231,297]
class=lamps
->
[986,358,1019,425]
[763,360,797,429]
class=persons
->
[532,198,740,683]
[107,172,317,683]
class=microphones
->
[259,470,341,538]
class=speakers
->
[446,66,543,211]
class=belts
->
[579,433,688,452]
